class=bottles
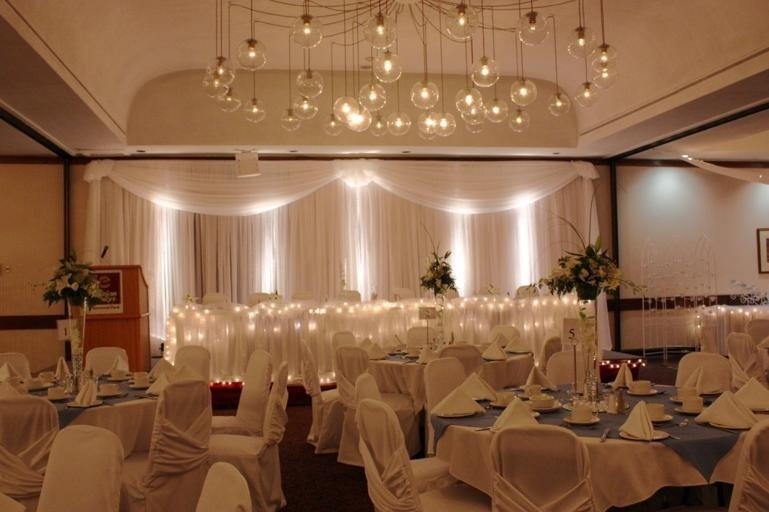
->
[592,384,631,415]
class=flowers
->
[31,256,109,310]
[532,185,648,303]
[416,221,461,296]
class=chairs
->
[2,286,767,410]
[355,399,491,511]
[116,380,212,512]
[34,425,124,511]
[354,372,460,497]
[488,424,599,511]
[734,417,769,512]
[197,461,253,510]
[212,351,273,438]
[1,394,60,509]
[209,362,290,509]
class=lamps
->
[199,0,617,141]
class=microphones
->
[100,245,109,257]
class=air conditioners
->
[231,147,261,180]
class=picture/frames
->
[755,227,769,274]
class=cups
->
[677,387,697,399]
[567,401,593,422]
[24,369,150,393]
[646,403,665,419]
[682,395,702,409]
[495,392,514,404]
[630,381,651,393]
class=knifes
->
[600,428,610,443]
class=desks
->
[434,382,769,511]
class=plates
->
[669,395,704,402]
[673,407,705,414]
[510,384,562,412]
[67,400,104,409]
[437,413,473,419]
[489,401,507,408]
[562,415,601,425]
[367,341,530,362]
[41,395,70,401]
[627,388,659,396]
[652,415,675,422]
[619,429,671,441]
[710,413,769,430]
